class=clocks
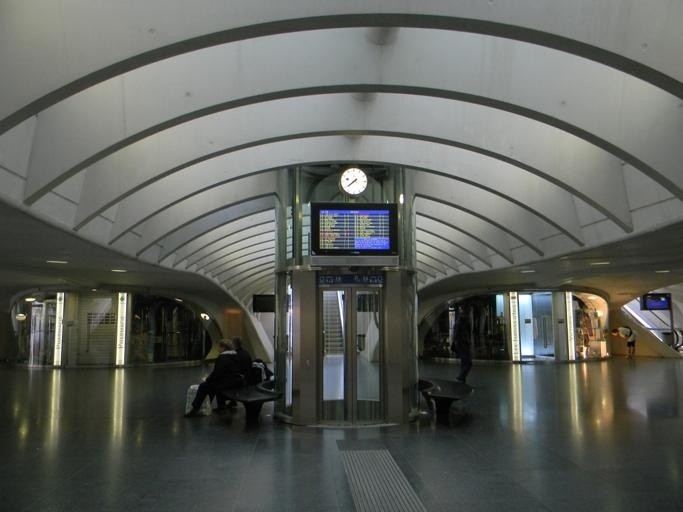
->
[335,165,368,196]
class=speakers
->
[309,255,400,266]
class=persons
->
[451,309,472,383]
[184,335,252,417]
[611,325,637,357]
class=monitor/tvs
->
[253,294,288,312]
[310,201,399,256]
[640,292,672,310]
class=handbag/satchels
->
[185,384,211,417]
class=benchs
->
[225,378,277,435]
[417,374,475,425]
